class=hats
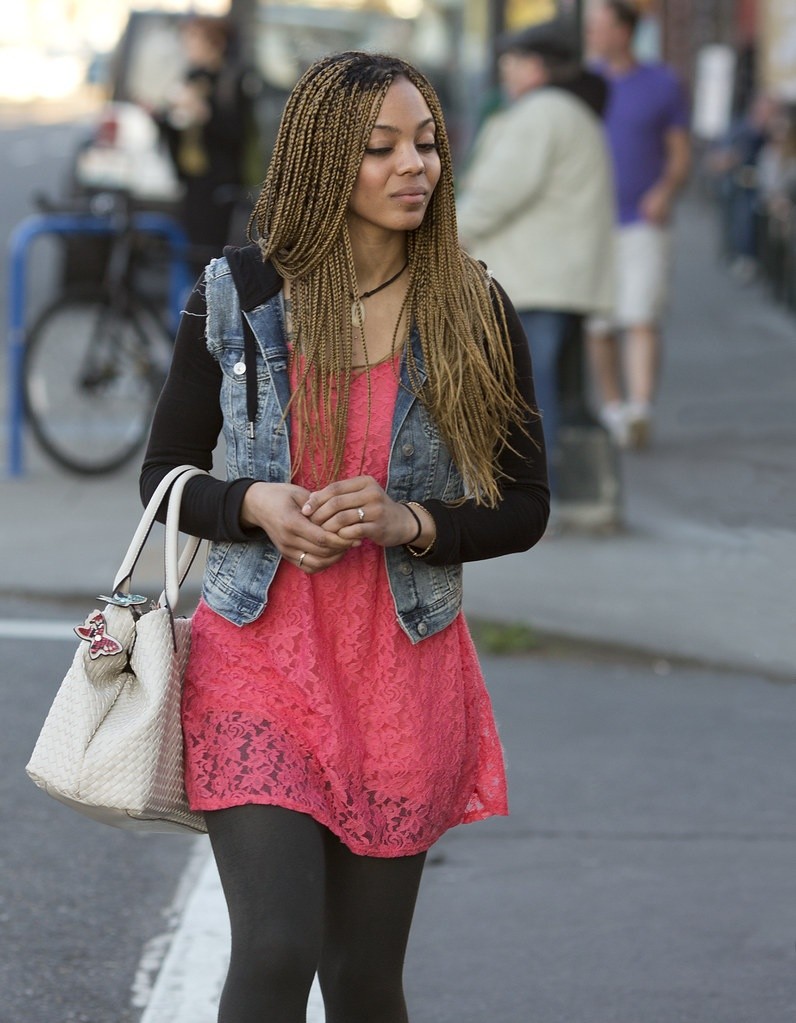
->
[480,24,573,58]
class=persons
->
[153,11,258,274]
[138,52,553,1023]
[576,0,692,450]
[454,30,623,534]
[707,97,796,273]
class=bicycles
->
[22,181,222,474]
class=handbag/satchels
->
[24,464,212,835]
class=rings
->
[358,507,364,521]
[299,552,307,566]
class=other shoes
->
[607,408,635,453]
[627,409,651,451]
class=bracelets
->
[401,503,422,544]
[408,501,437,558]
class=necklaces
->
[341,258,409,328]
